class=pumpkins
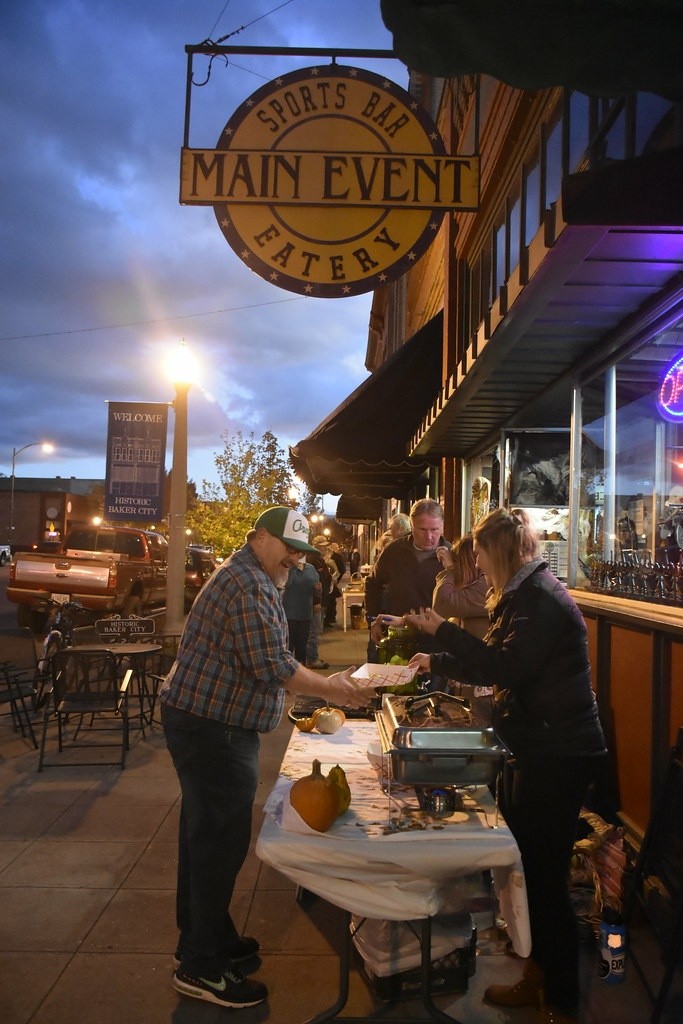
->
[291,758,350,833]
[295,700,345,734]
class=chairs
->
[0,626,177,772]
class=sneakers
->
[172,965,267,1007]
[173,936,260,967]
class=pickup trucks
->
[0,545,10,568]
[7,526,170,633]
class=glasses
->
[279,538,306,558]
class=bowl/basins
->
[348,583,361,589]
[464,784,489,800]
[367,742,393,785]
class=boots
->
[534,1008,577,1024]
[485,958,546,1006]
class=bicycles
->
[31,595,95,711]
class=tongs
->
[403,690,471,721]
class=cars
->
[34,540,62,553]
[183,547,219,599]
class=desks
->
[253,720,531,1024]
[343,592,366,632]
[61,643,164,741]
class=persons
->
[406,509,606,1024]
[280,499,490,669]
[159,507,372,1009]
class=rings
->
[345,703,350,707]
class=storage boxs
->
[349,663,419,688]
[370,926,478,1006]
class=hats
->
[253,506,321,557]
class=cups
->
[346,586,351,590]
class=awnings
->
[288,308,444,524]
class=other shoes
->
[305,659,330,669]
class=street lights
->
[163,338,197,648]
[10,441,54,545]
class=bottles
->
[598,906,627,984]
[367,618,418,695]
